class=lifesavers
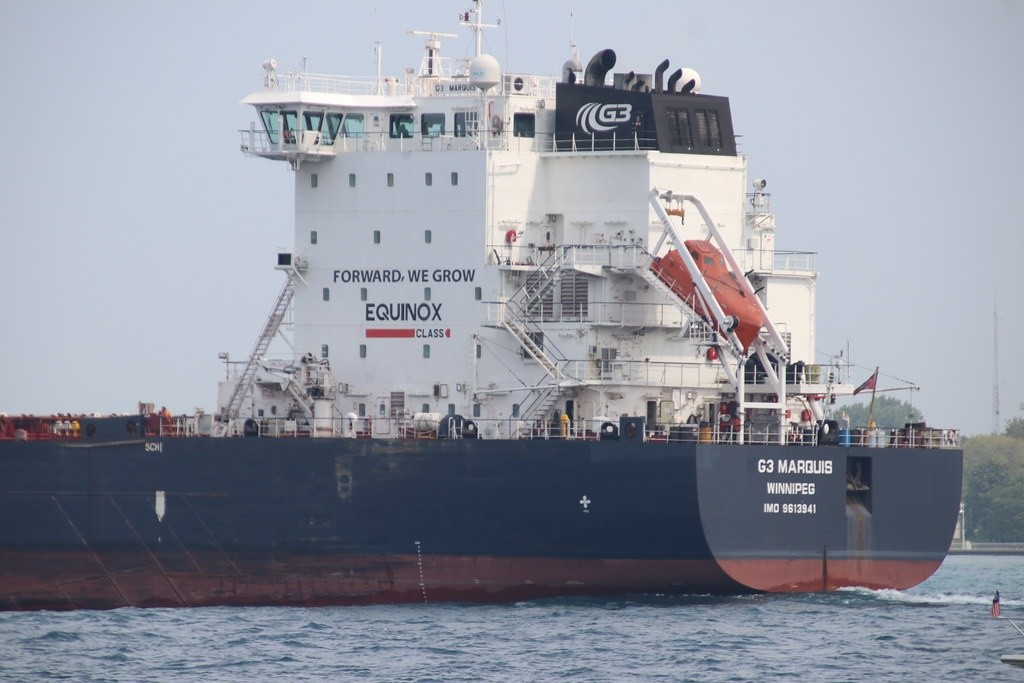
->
[506,229,517,242]
[462,420,480,442]
[947,428,956,445]
[708,348,718,360]
[126,420,136,432]
[599,421,619,439]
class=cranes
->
[991,284,1001,433]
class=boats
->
[986,591,1024,665]
[0,0,965,611]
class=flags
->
[854,371,878,396]
